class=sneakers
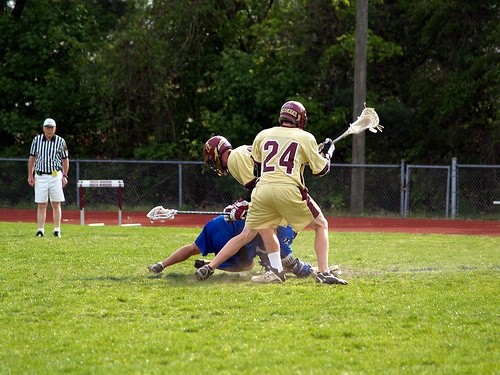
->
[315,272,348,285]
[251,267,287,283]
[147,262,164,274]
[194,259,204,268]
[195,264,215,280]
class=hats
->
[43,118,56,127]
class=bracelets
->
[63,174,68,178]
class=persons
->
[204,135,285,282]
[27,118,70,238]
[147,215,315,279]
[195,101,347,285]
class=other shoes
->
[36,229,44,237]
[53,231,61,238]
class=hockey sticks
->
[284,264,342,278]
[332,102,384,144]
[146,205,231,224]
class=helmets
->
[204,135,233,173]
[279,100,308,130]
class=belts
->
[37,171,52,175]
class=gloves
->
[229,200,249,222]
[258,260,272,271]
[319,137,335,159]
[290,258,313,278]
[224,197,244,222]
